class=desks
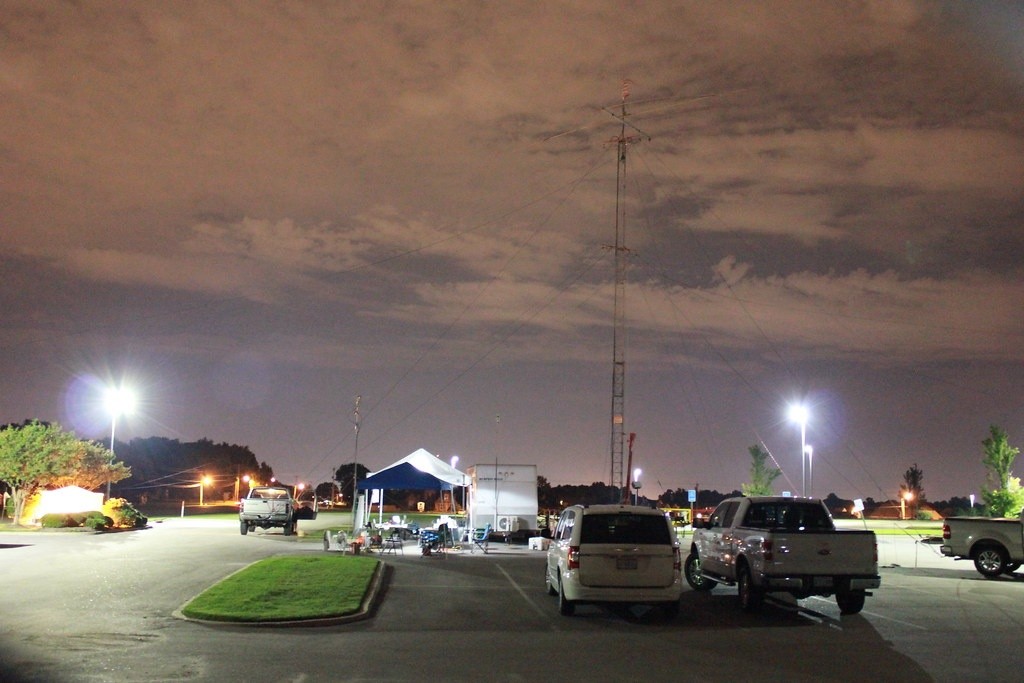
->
[374,522,407,555]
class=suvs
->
[544,503,683,617]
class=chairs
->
[472,524,491,554]
[336,515,413,556]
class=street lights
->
[791,405,807,498]
[805,444,813,497]
[101,388,127,501]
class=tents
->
[355,448,472,548]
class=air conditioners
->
[494,516,519,532]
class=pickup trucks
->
[940,509,1024,577]
[237,485,319,536]
[684,495,882,615]
